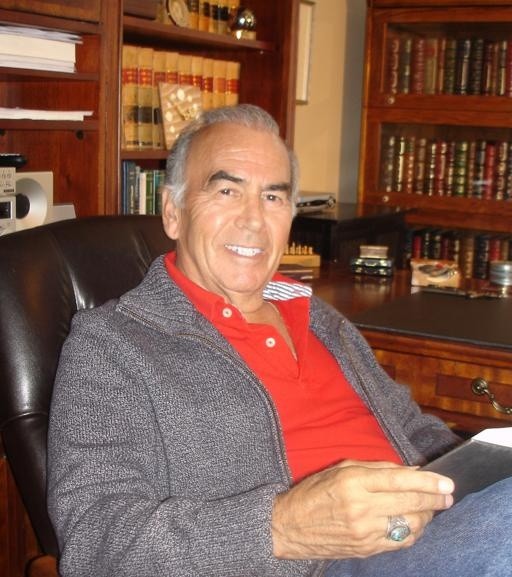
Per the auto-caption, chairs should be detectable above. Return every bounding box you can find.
[0,216,177,561]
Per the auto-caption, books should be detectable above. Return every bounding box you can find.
[382,135,512,199]
[119,45,240,149]
[401,225,512,280]
[157,0,240,38]
[415,427,512,517]
[389,35,511,98]
[120,160,166,217]
[0,34,76,63]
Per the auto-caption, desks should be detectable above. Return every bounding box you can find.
[293,202,417,281]
[300,274,512,435]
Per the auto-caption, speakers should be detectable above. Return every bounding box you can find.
[16,171,54,234]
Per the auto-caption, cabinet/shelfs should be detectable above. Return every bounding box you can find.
[2,0,112,236]
[112,2,298,234]
[361,2,512,292]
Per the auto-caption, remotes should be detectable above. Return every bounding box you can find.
[0,153,27,166]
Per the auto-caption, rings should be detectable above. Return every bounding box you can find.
[386,515,410,542]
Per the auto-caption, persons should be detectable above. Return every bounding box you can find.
[45,104,512,577]
